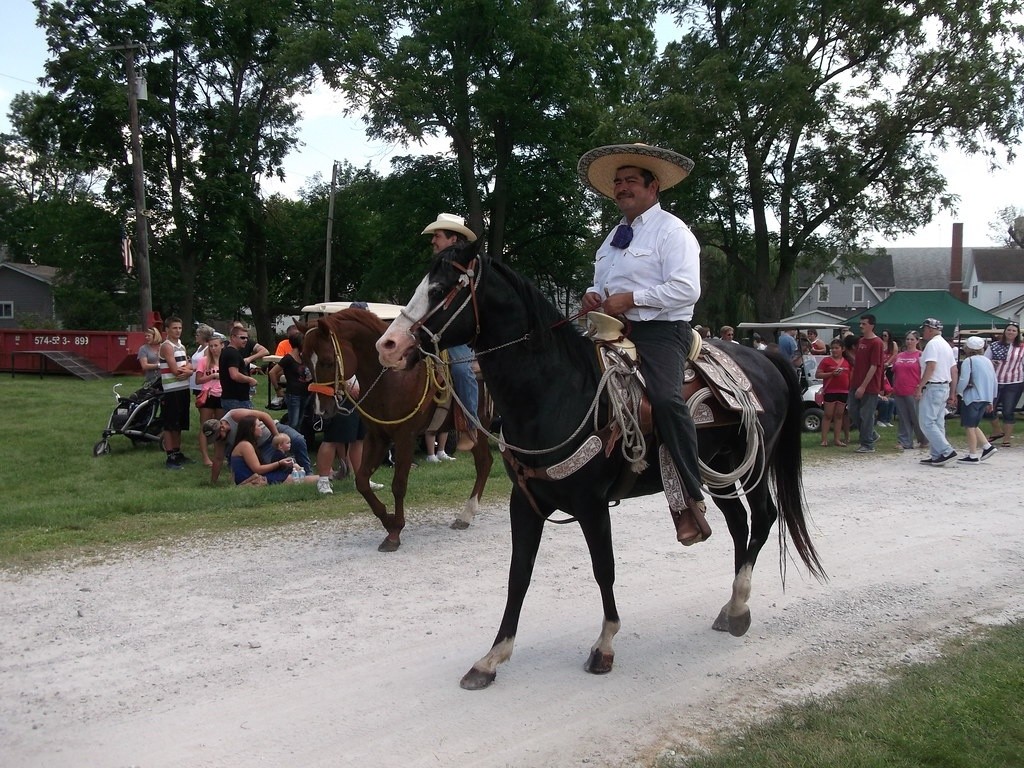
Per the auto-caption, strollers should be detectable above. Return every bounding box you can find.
[93,374,166,456]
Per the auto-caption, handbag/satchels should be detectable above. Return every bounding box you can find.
[815,387,825,407]
[238,473,267,488]
[195,387,211,408]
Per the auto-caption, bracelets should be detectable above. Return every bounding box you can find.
[157,362,161,368]
[277,460,281,468]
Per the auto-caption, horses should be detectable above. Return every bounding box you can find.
[373,234,832,692]
[291,305,497,556]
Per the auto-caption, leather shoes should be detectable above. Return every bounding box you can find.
[677,503,706,542]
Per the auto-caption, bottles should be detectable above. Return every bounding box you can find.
[299,467,306,483]
[291,467,300,486]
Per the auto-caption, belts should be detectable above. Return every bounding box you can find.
[927,381,949,384]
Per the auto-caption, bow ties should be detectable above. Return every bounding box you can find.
[609,224,633,249]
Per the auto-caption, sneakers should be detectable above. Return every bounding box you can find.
[931,449,959,466]
[354,478,385,492]
[980,445,998,461]
[317,477,334,494]
[920,458,931,465]
[957,455,979,465]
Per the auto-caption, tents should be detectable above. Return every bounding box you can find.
[834,290,1020,340]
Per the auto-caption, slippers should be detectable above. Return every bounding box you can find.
[1002,442,1011,447]
[987,432,1005,442]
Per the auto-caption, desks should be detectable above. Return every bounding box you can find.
[263,355,284,410]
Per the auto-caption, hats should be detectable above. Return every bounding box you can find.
[919,317,944,331]
[577,142,696,200]
[966,336,985,351]
[421,213,477,242]
[202,419,221,444]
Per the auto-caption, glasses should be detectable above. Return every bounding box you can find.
[235,336,248,339]
[727,334,734,336]
[808,332,813,335]
[706,336,713,339]
[882,335,886,337]
[1008,321,1019,326]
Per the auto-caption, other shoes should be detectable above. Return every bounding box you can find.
[176,452,197,464]
[853,444,875,452]
[877,420,887,427]
[820,440,828,447]
[333,457,348,481]
[166,454,183,470]
[920,442,929,447]
[885,422,894,427]
[896,443,914,449]
[425,454,442,463]
[834,441,846,446]
[437,452,456,461]
[856,430,880,444]
[456,428,478,451]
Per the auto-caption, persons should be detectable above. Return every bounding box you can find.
[694,313,1024,466]
[575,142,707,541]
[424,430,457,463]
[420,212,480,453]
[137,301,386,496]
